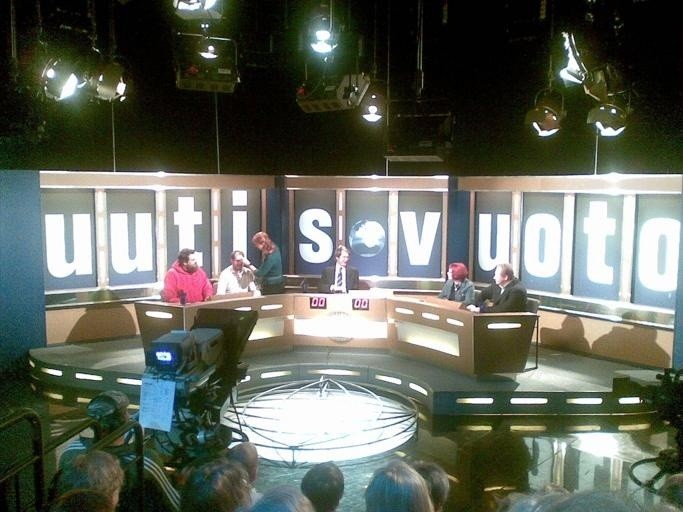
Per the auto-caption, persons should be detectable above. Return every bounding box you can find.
[213,251,256,296]
[47,390,180,511]
[317,245,359,293]
[436,263,474,305]
[180,442,682,510]
[466,263,529,313]
[159,247,212,304]
[238,232,285,295]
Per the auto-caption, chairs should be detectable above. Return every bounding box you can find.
[524,298,542,370]
[212,282,219,295]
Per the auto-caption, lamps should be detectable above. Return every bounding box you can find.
[558,18,629,94]
[363,95,384,121]
[172,1,225,26]
[305,2,343,57]
[527,87,567,137]
[298,71,372,112]
[173,32,240,92]
[586,99,635,138]
[87,41,129,100]
[29,37,79,102]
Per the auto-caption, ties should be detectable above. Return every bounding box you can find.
[337,268,343,293]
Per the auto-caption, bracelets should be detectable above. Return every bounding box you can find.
[246,263,251,268]
